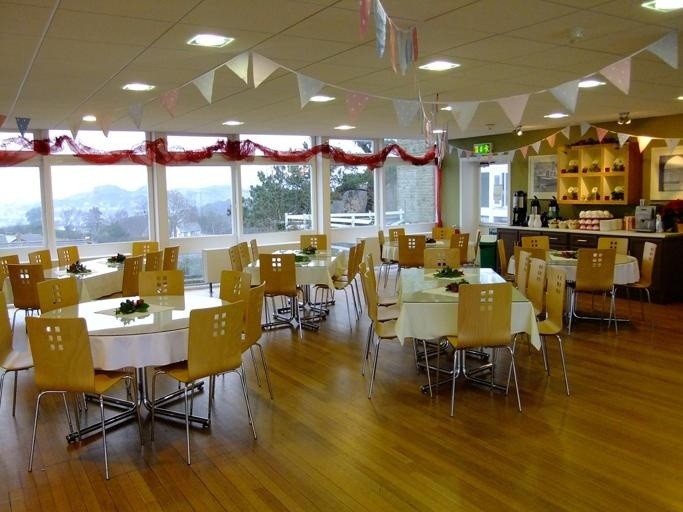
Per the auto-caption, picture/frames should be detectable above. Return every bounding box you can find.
[650,146,683,201]
[526,155,557,199]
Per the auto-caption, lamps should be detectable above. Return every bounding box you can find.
[618,112,631,124]
[512,125,523,136]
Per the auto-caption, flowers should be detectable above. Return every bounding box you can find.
[292,246,318,262]
[65,260,92,275]
[110,255,125,265]
[568,158,625,200]
[434,262,463,276]
[445,279,469,291]
[427,236,437,244]
[115,298,148,313]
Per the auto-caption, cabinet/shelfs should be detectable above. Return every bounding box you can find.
[629,237,682,305]
[557,141,642,205]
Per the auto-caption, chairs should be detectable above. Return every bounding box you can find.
[434,281,523,416]
[565,248,615,336]
[93,246,181,300]
[577,237,626,311]
[205,282,271,420]
[28,250,51,269]
[37,277,135,418]
[132,242,158,254]
[507,250,574,399]
[378,230,549,294]
[134,266,187,388]
[23,315,144,478]
[360,252,435,401]
[1,294,85,431]
[231,238,289,328]
[1,256,41,317]
[7,264,45,336]
[151,298,261,465]
[56,245,80,267]
[602,241,658,330]
[311,241,368,333]
[193,270,249,426]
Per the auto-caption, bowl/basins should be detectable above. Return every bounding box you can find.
[558,223,577,229]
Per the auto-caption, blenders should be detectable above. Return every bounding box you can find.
[511,190,560,226]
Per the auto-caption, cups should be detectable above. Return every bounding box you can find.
[579,210,612,230]
